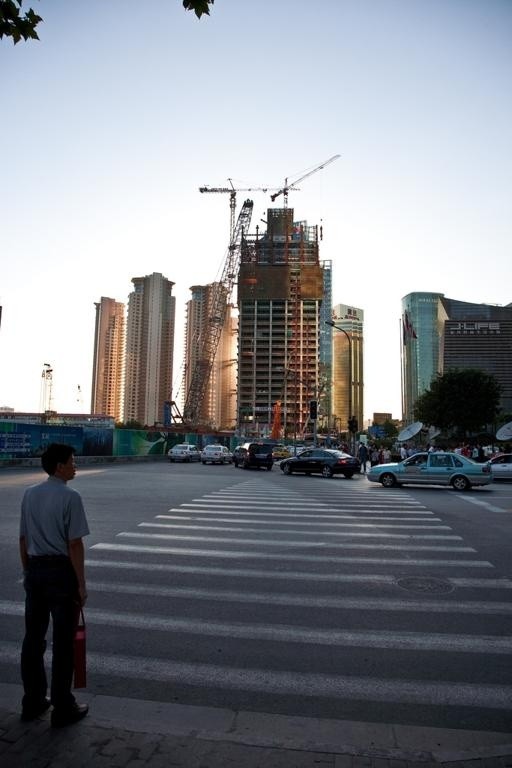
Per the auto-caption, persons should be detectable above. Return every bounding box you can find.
[328,440,488,473]
[19,444,91,728]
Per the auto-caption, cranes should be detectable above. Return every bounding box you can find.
[163,154,341,433]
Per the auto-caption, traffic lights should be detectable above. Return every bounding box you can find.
[310,402,317,419]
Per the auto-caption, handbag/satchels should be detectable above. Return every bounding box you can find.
[74,607,87,688]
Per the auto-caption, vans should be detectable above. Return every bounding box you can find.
[235,442,272,470]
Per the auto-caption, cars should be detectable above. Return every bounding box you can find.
[280,448,361,478]
[272,447,293,461]
[367,447,512,491]
[167,442,239,464]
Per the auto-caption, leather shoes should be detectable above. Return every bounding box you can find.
[50,702,89,728]
[20,697,53,722]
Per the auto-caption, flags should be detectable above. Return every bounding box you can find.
[402,311,417,346]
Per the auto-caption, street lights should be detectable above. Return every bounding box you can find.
[325,322,354,442]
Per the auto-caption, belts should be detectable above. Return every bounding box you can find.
[26,555,68,564]
